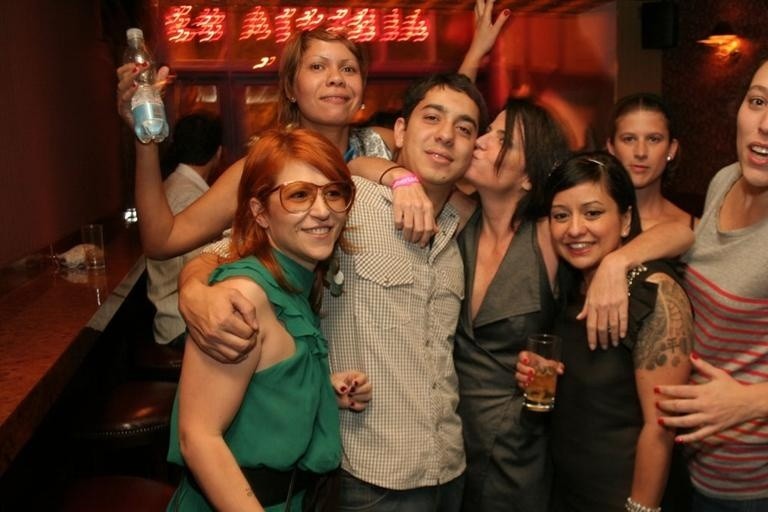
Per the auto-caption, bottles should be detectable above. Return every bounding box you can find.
[125,27,171,146]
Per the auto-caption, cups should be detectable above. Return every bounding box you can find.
[78,225,107,270]
[523,334,566,410]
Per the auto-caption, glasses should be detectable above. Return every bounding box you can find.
[259,180,355,214]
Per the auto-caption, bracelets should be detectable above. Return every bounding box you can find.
[373,165,409,178]
[387,176,425,185]
[622,496,664,512]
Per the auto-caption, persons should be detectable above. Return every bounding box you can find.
[161,142,371,511]
[148,109,237,369]
[176,69,489,512]
[652,45,768,511]
[345,100,696,510]
[113,1,512,261]
[512,155,699,511]
[601,87,703,241]
